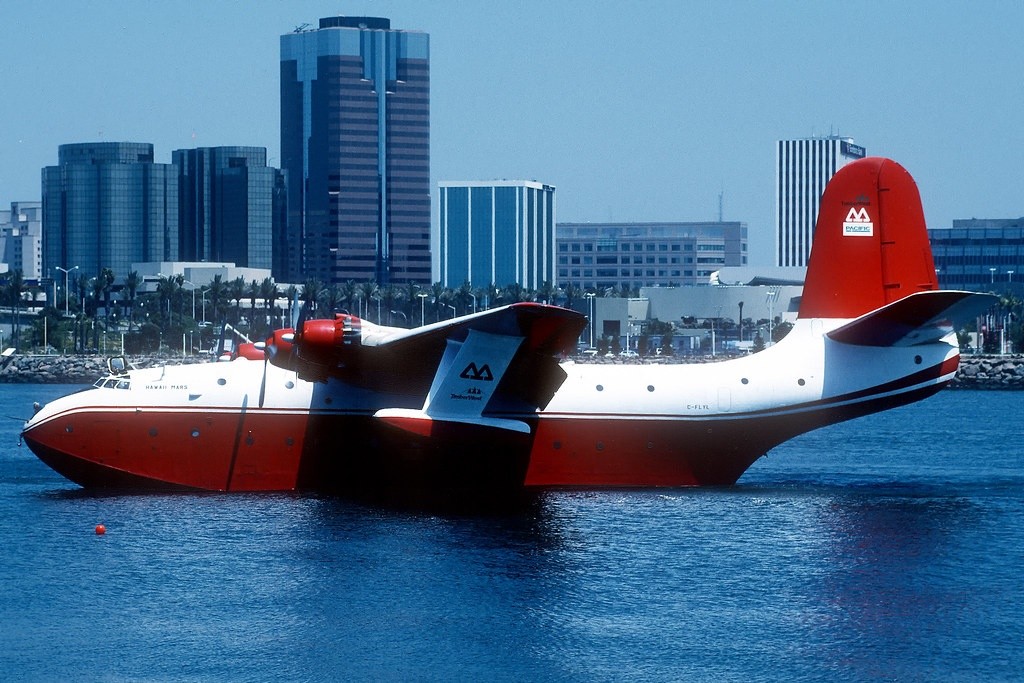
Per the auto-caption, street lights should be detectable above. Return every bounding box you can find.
[52,263,1016,360]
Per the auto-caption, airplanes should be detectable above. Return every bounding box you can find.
[18,155,978,500]
